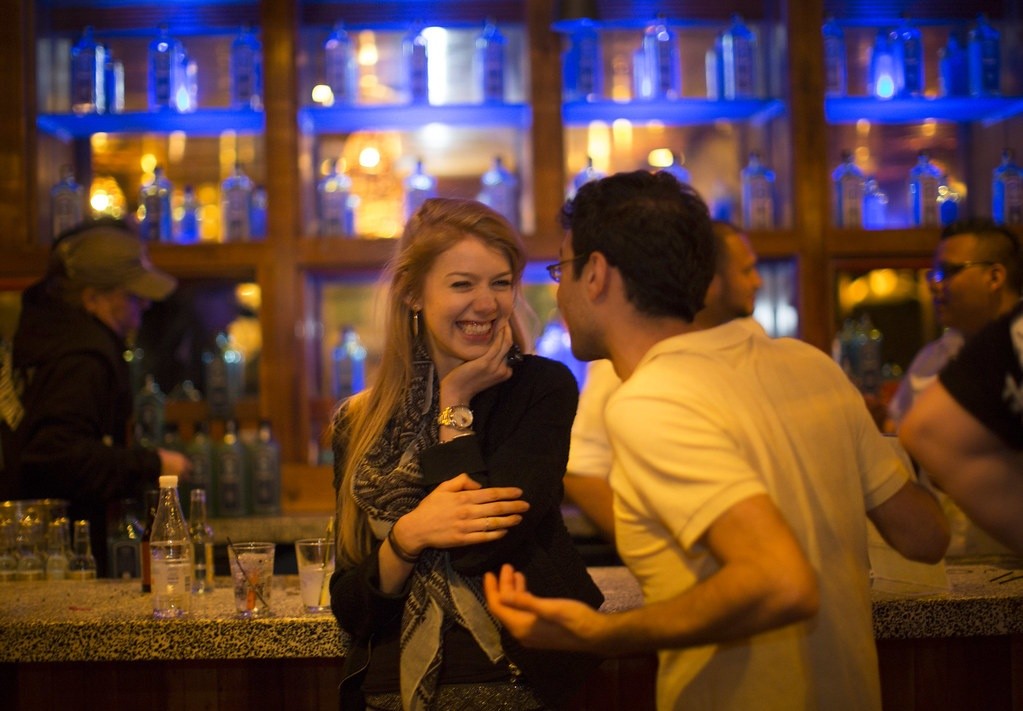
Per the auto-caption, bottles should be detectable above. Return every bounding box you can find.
[107,475,214,621]
[328,326,368,400]
[136,331,281,518]
[47,150,781,245]
[70,14,786,115]
[0,508,97,582]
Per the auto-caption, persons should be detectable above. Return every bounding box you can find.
[564,220,762,545]
[329,196,605,711]
[483,169,953,711]
[884,216,1023,555]
[0,219,195,577]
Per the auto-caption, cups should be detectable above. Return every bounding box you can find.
[226,542,276,619]
[295,537,336,615]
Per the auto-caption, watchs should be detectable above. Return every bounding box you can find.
[436,403,474,429]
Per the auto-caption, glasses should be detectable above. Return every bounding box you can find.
[927,258,993,282]
[547,252,586,284]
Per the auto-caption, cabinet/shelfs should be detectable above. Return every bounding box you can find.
[0,1,1023,510]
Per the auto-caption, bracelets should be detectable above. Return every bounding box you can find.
[387,520,420,562]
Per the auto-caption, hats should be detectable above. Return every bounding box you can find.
[47,227,176,299]
